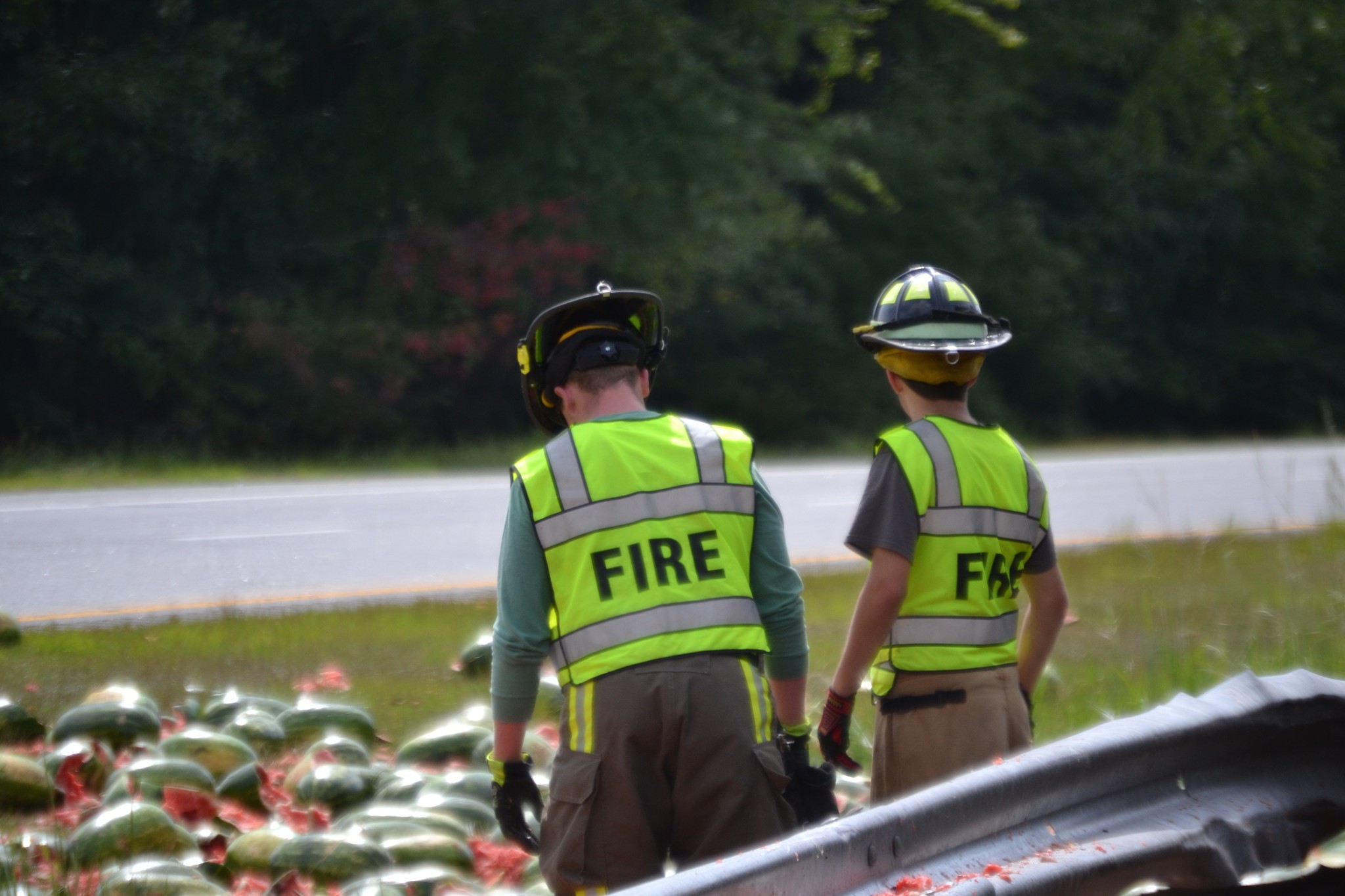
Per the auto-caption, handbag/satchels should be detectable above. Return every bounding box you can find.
[777,733,840,828]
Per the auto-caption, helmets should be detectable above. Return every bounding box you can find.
[853,265,1012,364]
[517,281,669,436]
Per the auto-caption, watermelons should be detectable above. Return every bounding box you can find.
[0,630,849,896]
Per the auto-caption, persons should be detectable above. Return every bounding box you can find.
[486,289,812,895]
[816,266,1070,805]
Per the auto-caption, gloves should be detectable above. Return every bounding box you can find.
[817,687,864,778]
[1019,685,1035,728]
[486,750,545,856]
[775,718,811,767]
